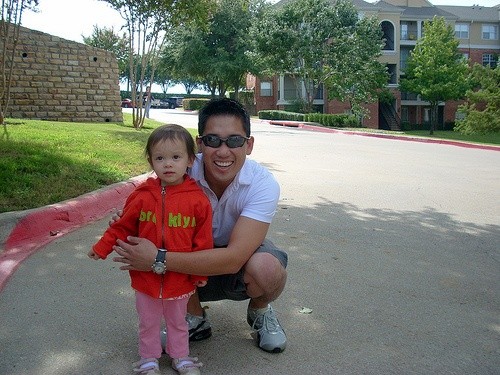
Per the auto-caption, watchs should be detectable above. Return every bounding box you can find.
[151,249,167,274]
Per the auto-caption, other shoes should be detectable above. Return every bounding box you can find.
[133,358,162,375]
[172,357,203,375]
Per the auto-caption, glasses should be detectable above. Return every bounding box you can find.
[198,134,248,149]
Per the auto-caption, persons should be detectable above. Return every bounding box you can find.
[87,124,214,375]
[109,98,288,354]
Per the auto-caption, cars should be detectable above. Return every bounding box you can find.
[122,95,184,109]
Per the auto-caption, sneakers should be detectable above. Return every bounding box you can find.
[247,303,287,353]
[161,305,211,354]
